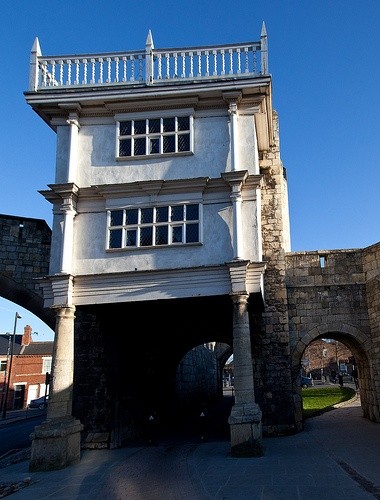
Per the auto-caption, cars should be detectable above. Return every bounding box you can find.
[29,395,50,411]
[300,375,314,389]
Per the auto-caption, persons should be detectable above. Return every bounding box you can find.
[351,366,359,390]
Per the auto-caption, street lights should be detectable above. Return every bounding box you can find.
[2,312,24,419]
